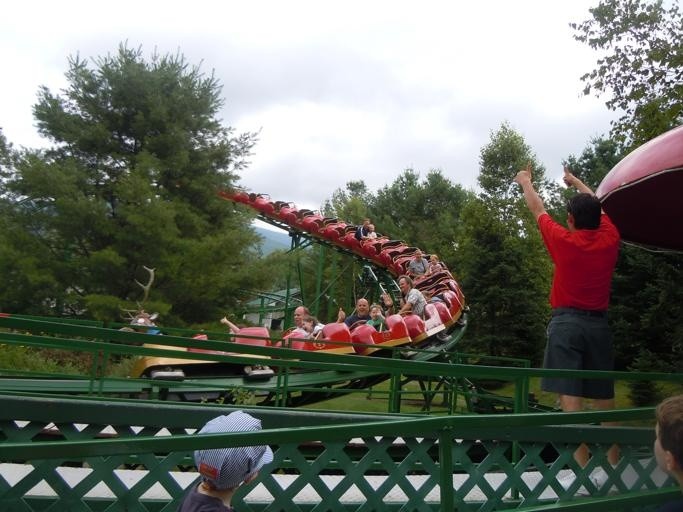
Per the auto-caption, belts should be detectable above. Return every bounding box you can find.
[552,307,604,317]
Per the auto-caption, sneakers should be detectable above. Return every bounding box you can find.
[555,465,617,495]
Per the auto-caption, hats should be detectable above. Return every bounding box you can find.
[194,410,274,490]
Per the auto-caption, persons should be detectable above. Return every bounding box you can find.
[177,411,274,510]
[654,397,679,489]
[221,219,440,338]
[515,162,619,496]
